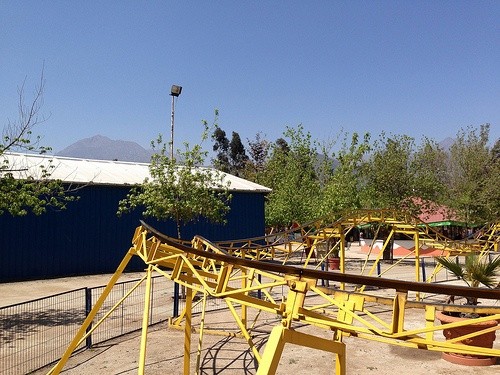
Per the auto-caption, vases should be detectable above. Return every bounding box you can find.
[328,247,340,270]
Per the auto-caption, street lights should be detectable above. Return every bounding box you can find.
[168,82,186,272]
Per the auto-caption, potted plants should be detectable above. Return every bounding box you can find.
[430,253,499,367]
